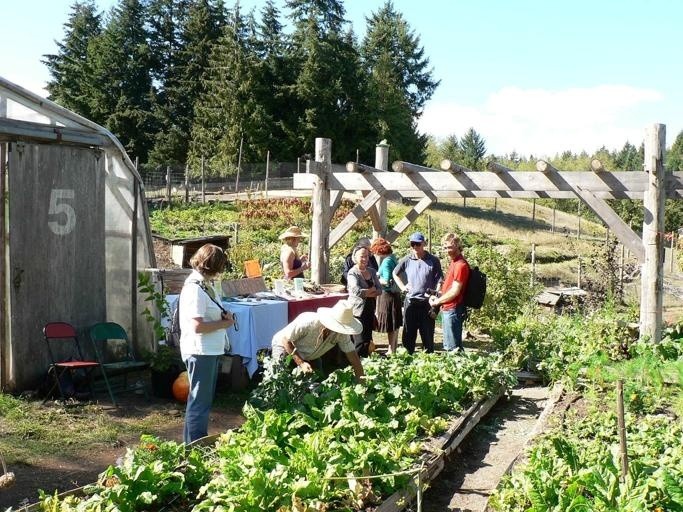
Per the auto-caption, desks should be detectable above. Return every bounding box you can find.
[221,289,349,379]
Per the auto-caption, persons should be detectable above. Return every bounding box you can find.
[278,226,309,280]
[391,233,443,356]
[370,237,403,354]
[345,246,382,359]
[270,298,365,381]
[427,233,470,350]
[340,236,380,286]
[177,243,236,447]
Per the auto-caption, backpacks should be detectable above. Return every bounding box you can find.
[463,259,486,309]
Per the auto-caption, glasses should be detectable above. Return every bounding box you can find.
[411,242,421,247]
[233,313,239,331]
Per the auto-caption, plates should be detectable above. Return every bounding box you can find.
[301,287,324,294]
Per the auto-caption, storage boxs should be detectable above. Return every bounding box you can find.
[216,353,243,393]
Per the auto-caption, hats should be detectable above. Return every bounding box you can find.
[279,226,305,239]
[317,300,363,335]
[410,232,424,242]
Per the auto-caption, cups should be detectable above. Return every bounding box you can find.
[293,277,304,301]
[274,280,284,296]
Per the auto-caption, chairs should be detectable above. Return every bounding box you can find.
[42,322,100,413]
[88,322,152,407]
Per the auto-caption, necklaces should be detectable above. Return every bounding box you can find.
[359,268,367,273]
